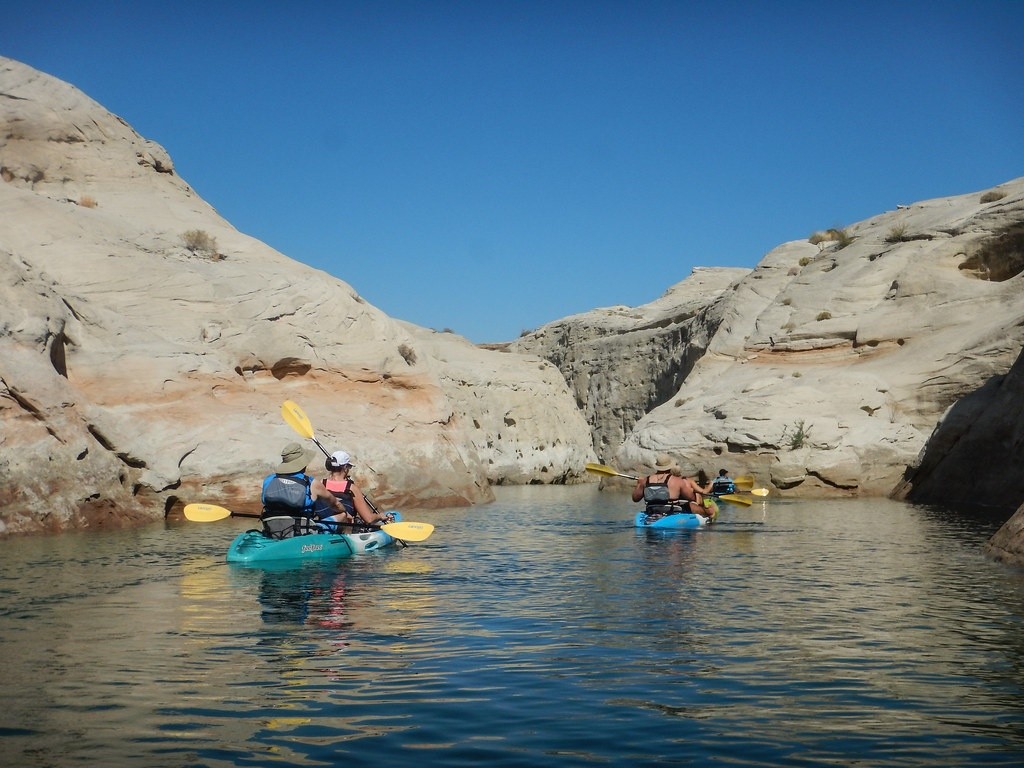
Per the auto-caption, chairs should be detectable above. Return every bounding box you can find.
[261,516,319,538]
[647,505,682,514]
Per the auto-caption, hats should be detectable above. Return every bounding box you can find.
[718,469,728,476]
[330,450,355,467]
[275,443,316,474]
[653,453,676,471]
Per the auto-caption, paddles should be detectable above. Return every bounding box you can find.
[281,399,408,547]
[585,462,752,509]
[696,474,754,489]
[737,488,769,497]
[182,502,435,542]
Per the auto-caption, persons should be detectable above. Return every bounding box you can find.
[710,469,741,496]
[261,442,354,535]
[632,454,718,519]
[321,451,389,533]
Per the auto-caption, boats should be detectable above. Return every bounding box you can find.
[226,511,401,569]
[634,510,715,531]
